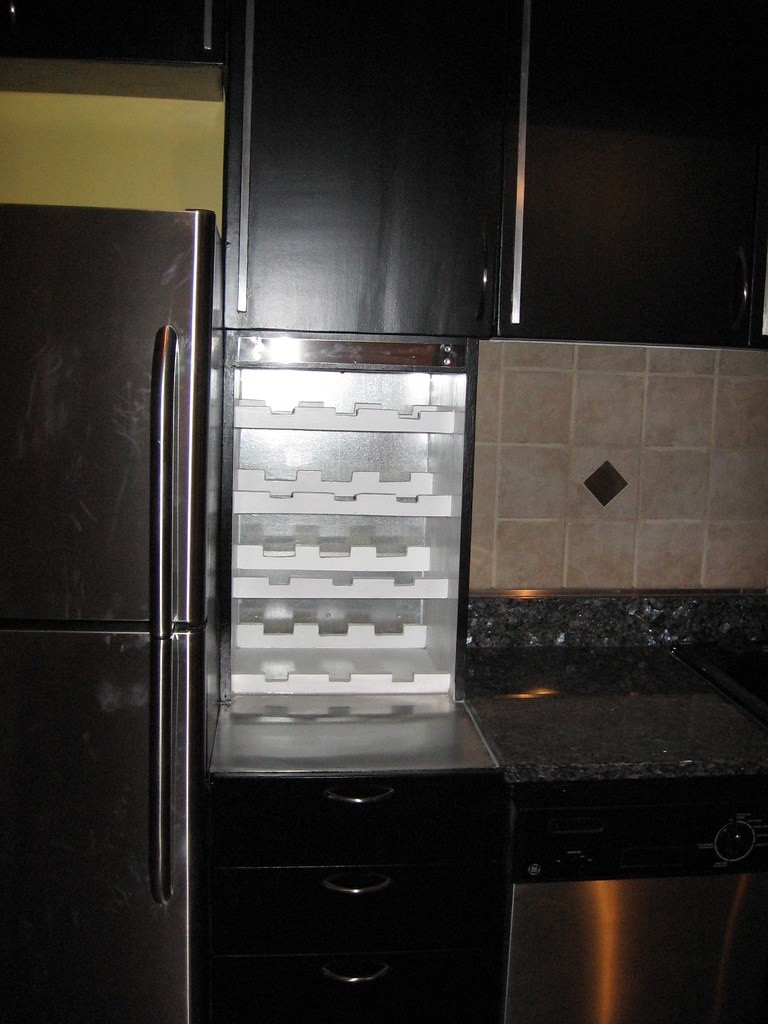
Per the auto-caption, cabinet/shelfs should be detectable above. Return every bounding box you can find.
[199,777,514,1023]
[0,0,768,346]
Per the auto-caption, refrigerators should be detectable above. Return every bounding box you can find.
[0,203,219,1024]
